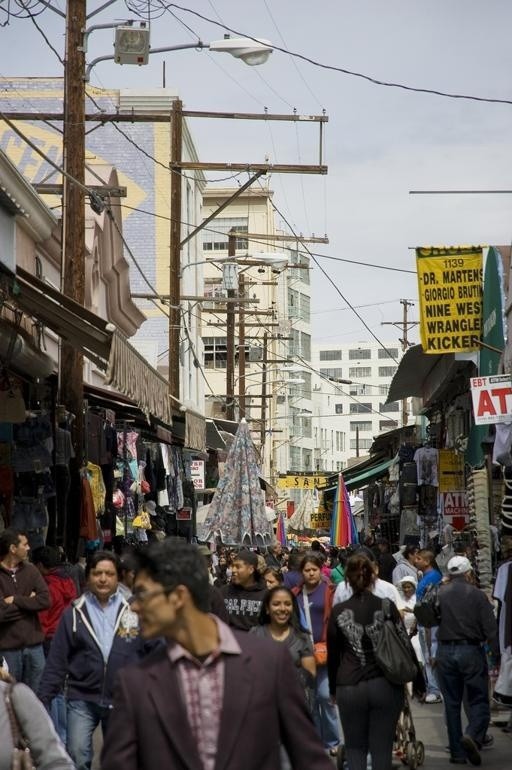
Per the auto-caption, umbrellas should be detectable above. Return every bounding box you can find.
[327,470,364,550]
[204,416,284,556]
[288,486,319,534]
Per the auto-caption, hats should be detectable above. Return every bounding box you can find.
[448,556,473,575]
[399,576,416,588]
[145,500,156,516]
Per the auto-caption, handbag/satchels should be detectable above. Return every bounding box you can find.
[133,512,152,529]
[130,471,150,496]
[313,641,326,664]
[12,747,36,770]
[364,599,417,686]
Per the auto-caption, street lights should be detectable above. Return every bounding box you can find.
[225,365,307,424]
[61,37,276,559]
[167,253,290,462]
[238,377,306,424]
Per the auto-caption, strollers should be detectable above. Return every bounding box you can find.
[335,684,424,770]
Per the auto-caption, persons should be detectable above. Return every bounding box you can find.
[1,529,442,770]
[435,555,501,768]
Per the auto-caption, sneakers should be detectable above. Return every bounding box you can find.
[445,734,493,765]
[425,693,442,703]
